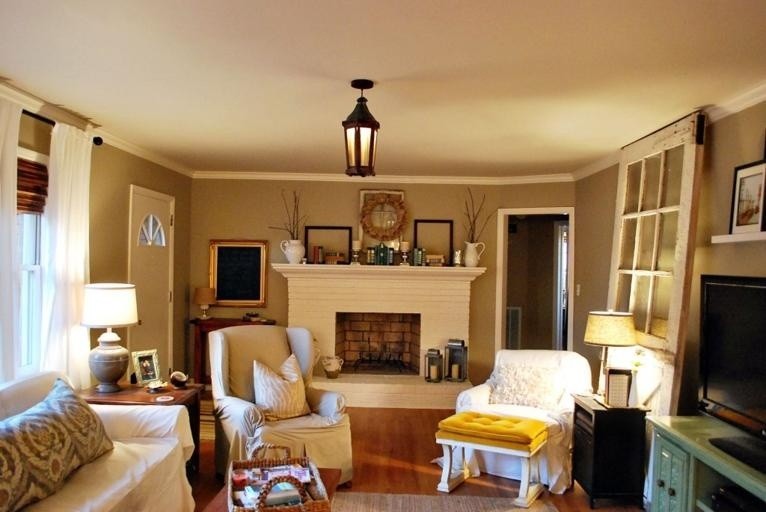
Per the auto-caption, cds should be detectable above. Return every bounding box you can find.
[156,395,174,402]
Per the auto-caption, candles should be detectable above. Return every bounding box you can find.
[429,363,439,381]
[351,240,361,251]
[449,362,459,380]
[400,241,410,252]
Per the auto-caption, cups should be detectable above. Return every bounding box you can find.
[313,246,324,263]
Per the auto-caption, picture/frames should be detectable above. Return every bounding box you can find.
[130,347,163,386]
[206,236,271,309]
[412,217,454,267]
[303,223,354,265]
[726,158,766,235]
[357,188,406,255]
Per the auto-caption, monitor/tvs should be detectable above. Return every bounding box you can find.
[697,274,766,475]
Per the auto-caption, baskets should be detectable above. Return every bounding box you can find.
[226,443,330,512]
[320,357,345,379]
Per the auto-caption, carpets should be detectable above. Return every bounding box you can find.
[325,487,562,511]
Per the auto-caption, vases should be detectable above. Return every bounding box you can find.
[463,240,487,267]
[278,239,307,264]
[321,355,346,381]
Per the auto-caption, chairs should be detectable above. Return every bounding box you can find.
[204,320,355,494]
[453,346,598,497]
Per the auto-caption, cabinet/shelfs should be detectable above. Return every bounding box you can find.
[566,388,652,508]
[639,408,766,511]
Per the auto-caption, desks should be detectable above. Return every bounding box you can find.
[185,314,277,400]
[74,380,206,480]
[202,460,344,510]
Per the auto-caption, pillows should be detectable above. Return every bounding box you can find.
[251,355,314,421]
[0,375,112,510]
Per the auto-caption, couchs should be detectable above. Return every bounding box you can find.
[0,368,201,510]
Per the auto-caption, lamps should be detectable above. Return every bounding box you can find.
[192,286,217,321]
[339,77,381,179]
[580,307,640,409]
[81,280,141,396]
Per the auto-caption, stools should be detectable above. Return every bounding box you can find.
[432,409,554,508]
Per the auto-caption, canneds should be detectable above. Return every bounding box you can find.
[366,247,375,265]
[313,245,323,264]
[389,248,393,264]
[413,248,426,266]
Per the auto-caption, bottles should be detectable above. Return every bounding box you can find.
[322,353,343,378]
[453,249,464,265]
